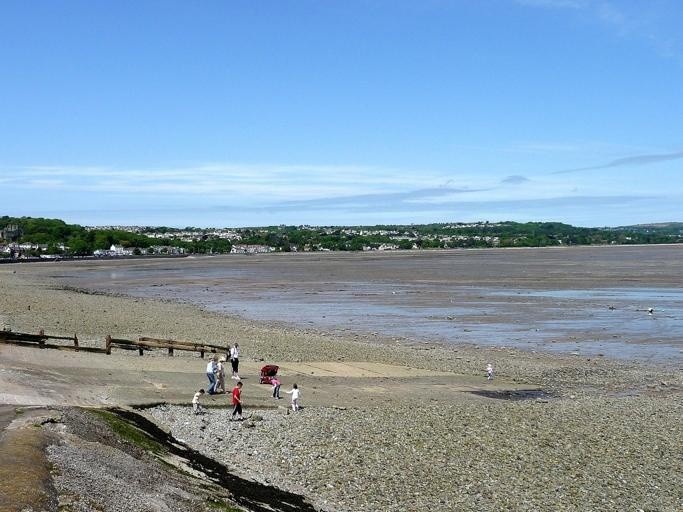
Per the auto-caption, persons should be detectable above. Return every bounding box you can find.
[228,381,245,421]
[268,378,280,400]
[205,355,218,395]
[229,342,241,380]
[191,389,205,412]
[284,382,301,411]
[485,363,493,380]
[213,359,229,394]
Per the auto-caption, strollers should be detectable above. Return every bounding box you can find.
[260,364,279,384]
